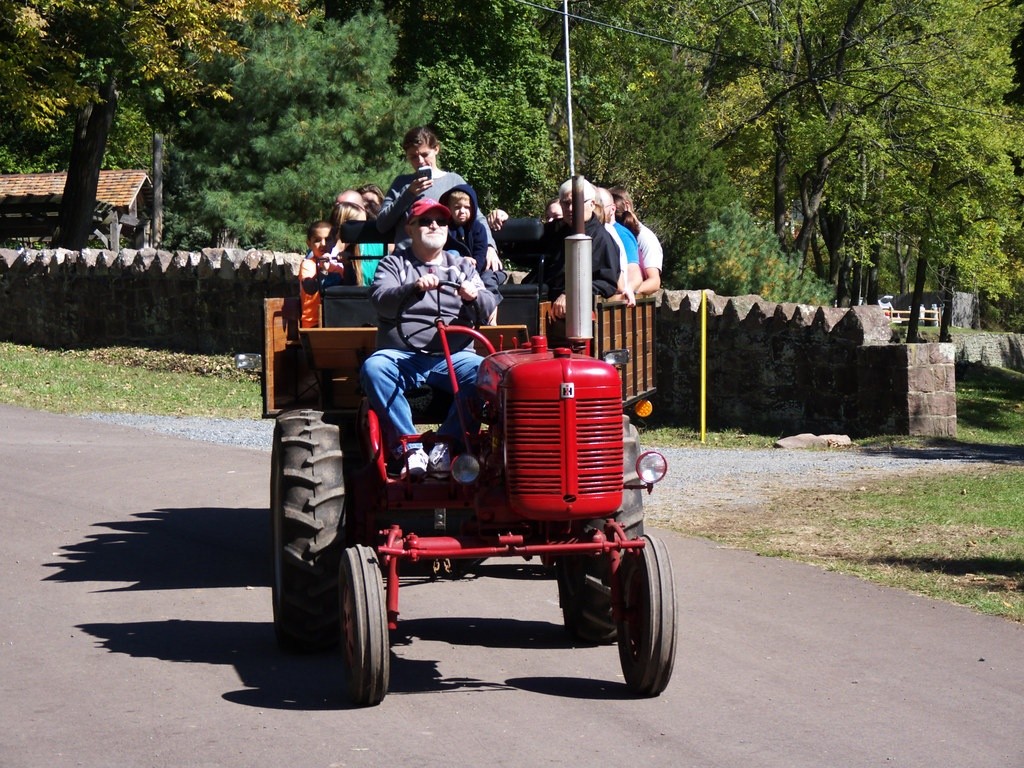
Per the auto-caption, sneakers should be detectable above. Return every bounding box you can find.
[428,442,452,479]
[400,447,430,481]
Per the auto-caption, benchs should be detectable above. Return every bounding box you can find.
[314,217,544,338]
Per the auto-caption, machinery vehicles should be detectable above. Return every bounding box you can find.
[256,172,683,707]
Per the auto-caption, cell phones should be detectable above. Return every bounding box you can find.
[416,166,432,181]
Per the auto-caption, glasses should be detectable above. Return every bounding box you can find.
[410,217,449,226]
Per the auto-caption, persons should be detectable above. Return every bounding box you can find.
[434,184,507,327]
[361,198,497,484]
[378,126,505,274]
[486,175,622,348]
[307,177,665,308]
[299,221,348,328]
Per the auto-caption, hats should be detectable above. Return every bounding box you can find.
[407,197,452,224]
